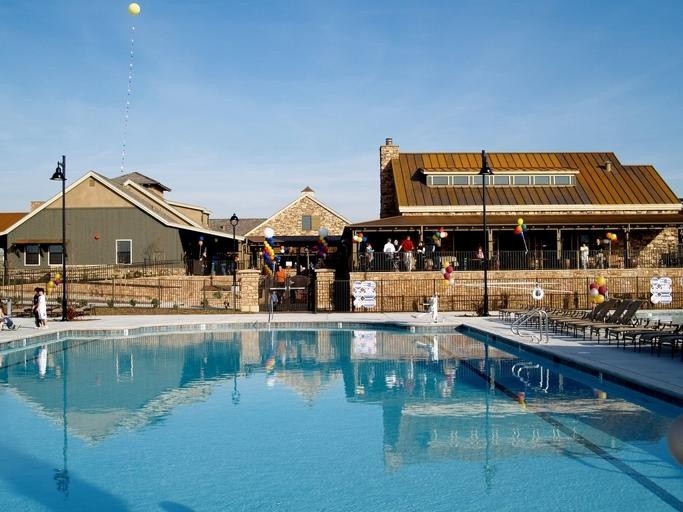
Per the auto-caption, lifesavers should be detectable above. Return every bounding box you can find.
[532,287,544,300]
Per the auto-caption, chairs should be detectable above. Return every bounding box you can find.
[550,299,645,339]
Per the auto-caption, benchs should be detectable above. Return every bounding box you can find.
[496,302,588,332]
[589,316,683,361]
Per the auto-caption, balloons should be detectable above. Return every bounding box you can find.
[589,276,607,303]
[603,233,619,244]
[263,228,330,280]
[128,3,140,16]
[353,233,367,242]
[231,254,239,262]
[433,227,448,241]
[46,272,63,295]
[215,238,218,242]
[514,218,527,234]
[199,236,204,246]
[441,261,454,286]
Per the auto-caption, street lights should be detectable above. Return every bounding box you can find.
[478,149,494,317]
[230,213,239,309]
[49,155,68,322]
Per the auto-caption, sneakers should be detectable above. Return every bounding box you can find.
[10,324,19,330]
[33,325,47,330]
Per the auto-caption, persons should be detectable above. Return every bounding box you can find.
[580,238,604,269]
[32,288,47,329]
[474,246,498,269]
[605,287,608,300]
[0,305,21,331]
[365,237,441,272]
[270,266,285,311]
[429,292,438,322]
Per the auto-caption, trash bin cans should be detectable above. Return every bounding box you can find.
[424,258,433,271]
[617,257,625,268]
[563,259,571,269]
[361,257,369,271]
[564,292,573,310]
